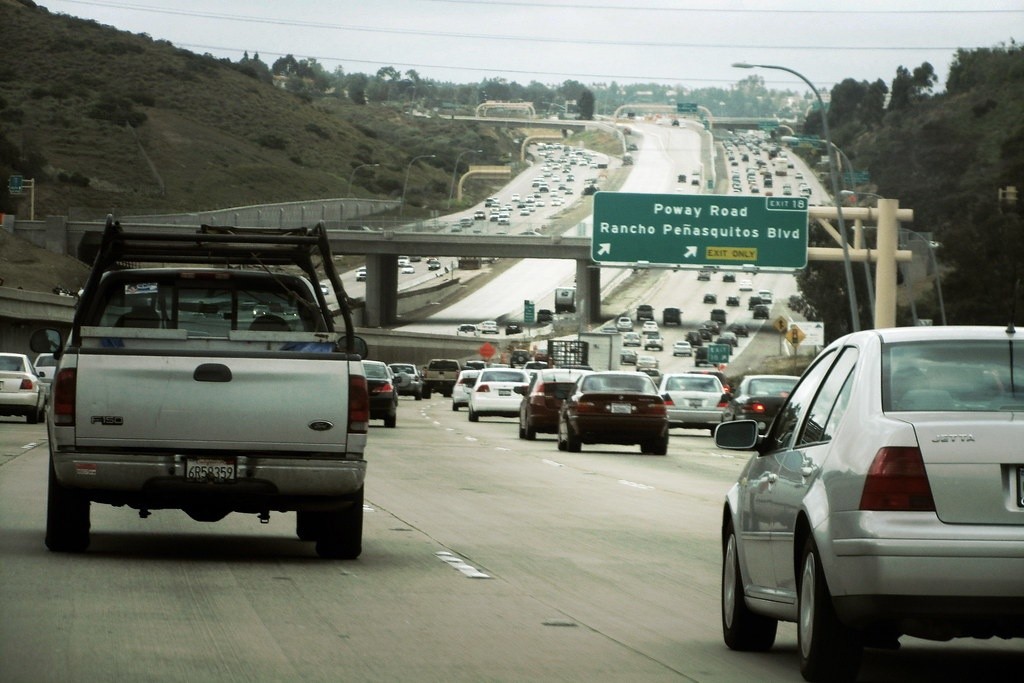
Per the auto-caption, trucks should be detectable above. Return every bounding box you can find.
[579,331,622,372]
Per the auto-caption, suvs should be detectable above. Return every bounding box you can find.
[387,363,423,400]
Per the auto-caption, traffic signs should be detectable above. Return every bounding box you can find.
[591,190,808,270]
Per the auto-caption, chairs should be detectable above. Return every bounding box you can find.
[113,311,159,329]
[247,315,292,332]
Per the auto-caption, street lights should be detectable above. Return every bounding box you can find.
[781,136,876,319]
[729,62,862,331]
[399,154,436,215]
[347,163,380,198]
[839,190,950,325]
[448,150,483,210]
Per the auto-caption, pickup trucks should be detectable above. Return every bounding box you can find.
[44,213,369,559]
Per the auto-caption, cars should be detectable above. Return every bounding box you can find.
[0,352,50,424]
[537,309,554,322]
[32,352,58,390]
[505,322,523,335]
[677,133,815,200]
[602,264,778,396]
[519,368,595,440]
[457,320,500,336]
[359,359,402,428]
[558,371,670,456]
[422,340,592,422]
[319,139,609,305]
[713,325,1023,683]
[720,373,802,434]
[656,373,736,440]
